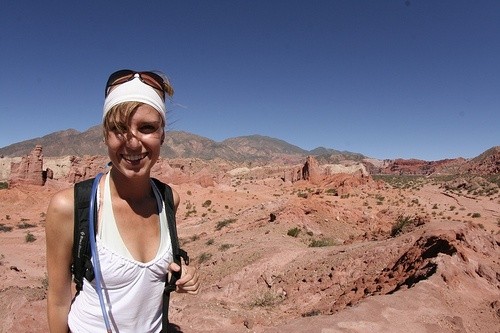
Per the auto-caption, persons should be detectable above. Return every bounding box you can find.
[44,69,201,333]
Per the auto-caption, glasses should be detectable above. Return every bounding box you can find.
[105,69,166,104]
